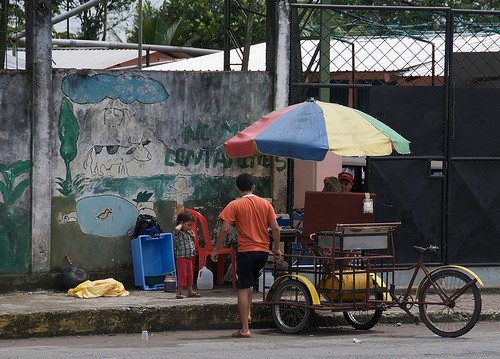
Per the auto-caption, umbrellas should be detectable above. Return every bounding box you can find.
[223,95,414,192]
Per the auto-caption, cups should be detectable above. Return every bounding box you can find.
[142,331,148,340]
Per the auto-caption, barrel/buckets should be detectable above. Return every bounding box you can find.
[164,272,176,293]
[258,270,274,293]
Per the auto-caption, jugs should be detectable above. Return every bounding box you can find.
[196,266,213,289]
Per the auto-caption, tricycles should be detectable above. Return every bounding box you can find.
[254,221,482,337]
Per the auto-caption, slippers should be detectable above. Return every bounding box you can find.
[231,328,252,338]
[176,292,183,299]
[235,314,251,324]
[186,292,201,298]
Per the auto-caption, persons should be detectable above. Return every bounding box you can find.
[210,172,281,339]
[321,171,354,192]
[174,210,202,299]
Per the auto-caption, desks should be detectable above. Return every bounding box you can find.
[263,229,298,302]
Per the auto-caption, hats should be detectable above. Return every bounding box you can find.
[338,172,353,183]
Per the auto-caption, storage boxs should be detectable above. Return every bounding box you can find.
[276,213,291,229]
[130,233,178,290]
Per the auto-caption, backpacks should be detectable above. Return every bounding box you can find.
[131,214,163,240]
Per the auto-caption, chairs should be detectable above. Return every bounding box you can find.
[184,208,236,289]
